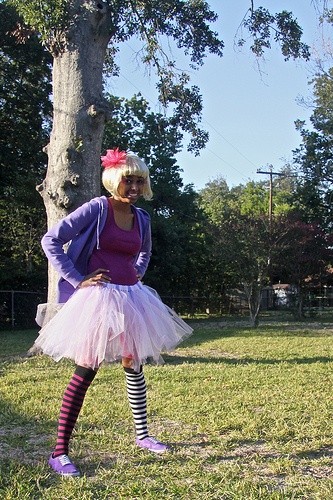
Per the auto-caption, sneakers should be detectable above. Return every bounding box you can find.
[49,452,80,477]
[137,437,170,453]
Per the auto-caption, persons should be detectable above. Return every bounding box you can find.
[28,148,195,477]
